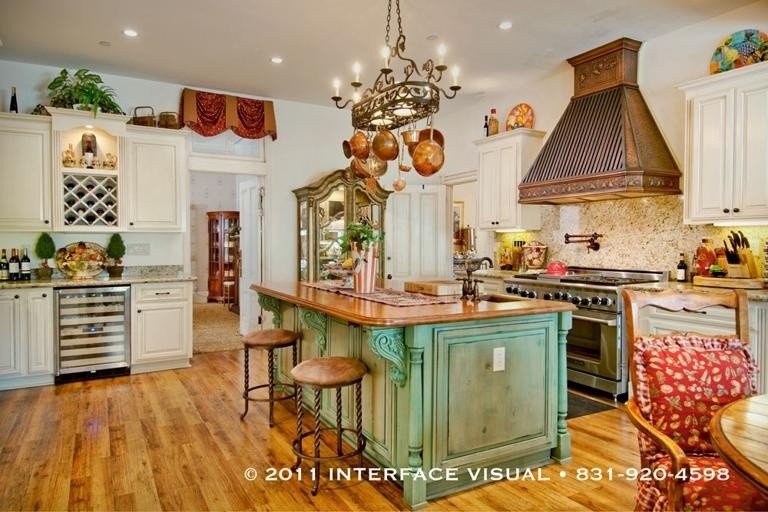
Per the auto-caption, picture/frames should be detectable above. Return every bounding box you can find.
[452,201,465,245]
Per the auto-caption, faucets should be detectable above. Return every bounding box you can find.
[456,257,494,301]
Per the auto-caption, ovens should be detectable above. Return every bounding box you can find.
[567,309,622,398]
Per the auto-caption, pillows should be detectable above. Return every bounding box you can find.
[628,331,761,463]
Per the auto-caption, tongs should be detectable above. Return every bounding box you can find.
[519,85,683,206]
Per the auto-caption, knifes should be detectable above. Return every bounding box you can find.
[721,229,750,264]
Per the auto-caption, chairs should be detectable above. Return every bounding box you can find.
[621,284,760,511]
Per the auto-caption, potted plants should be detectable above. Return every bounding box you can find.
[105,232,127,277]
[35,233,56,280]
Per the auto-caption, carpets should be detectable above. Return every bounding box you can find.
[565,387,617,419]
[191,301,247,356]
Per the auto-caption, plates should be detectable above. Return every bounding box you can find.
[506,103,535,130]
[57,242,108,278]
[710,28,768,75]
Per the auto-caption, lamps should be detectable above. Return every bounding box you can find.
[330,0,461,132]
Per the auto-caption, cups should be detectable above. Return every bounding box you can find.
[495,242,512,270]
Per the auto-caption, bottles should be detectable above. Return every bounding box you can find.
[9,86,18,114]
[677,252,687,281]
[689,252,702,282]
[488,109,499,136]
[65,182,116,225]
[511,240,525,270]
[0,247,32,281]
[481,116,488,138]
[84,140,93,168]
[699,239,716,274]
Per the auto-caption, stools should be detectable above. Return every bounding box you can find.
[237,329,302,429]
[289,355,370,495]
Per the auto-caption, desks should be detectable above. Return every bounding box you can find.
[250,282,577,511]
[710,393,768,496]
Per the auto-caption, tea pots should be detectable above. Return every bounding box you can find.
[61,142,75,167]
[102,151,116,170]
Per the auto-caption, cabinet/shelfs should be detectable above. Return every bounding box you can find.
[130,277,197,375]
[1,283,56,391]
[473,128,544,231]
[124,125,191,233]
[293,169,395,284]
[453,271,506,296]
[674,60,768,228]
[0,112,52,233]
[205,211,240,303]
[625,285,768,402]
[46,106,125,233]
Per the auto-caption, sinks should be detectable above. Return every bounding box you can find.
[478,293,531,303]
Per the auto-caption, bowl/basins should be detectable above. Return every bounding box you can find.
[523,246,547,268]
[547,262,568,272]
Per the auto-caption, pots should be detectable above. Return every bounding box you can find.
[342,110,445,179]
